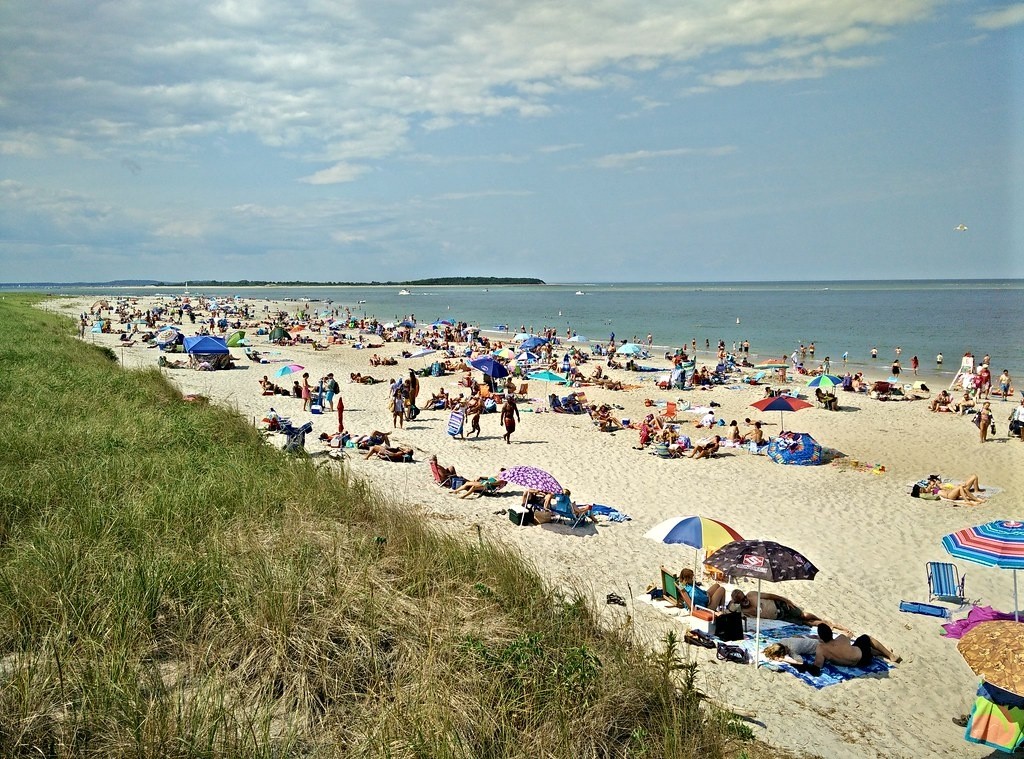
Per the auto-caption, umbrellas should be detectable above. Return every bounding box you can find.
[495,466,563,531]
[805,372,842,411]
[157,326,181,333]
[410,348,436,370]
[749,396,814,430]
[518,335,548,350]
[957,619,1024,699]
[942,519,1024,623]
[273,364,305,386]
[514,351,540,370]
[129,318,148,336]
[493,348,516,359]
[753,358,790,397]
[703,539,820,672]
[527,369,567,407]
[290,324,309,340]
[471,358,509,393]
[467,355,492,375]
[615,342,642,371]
[567,334,589,358]
[513,333,532,344]
[337,397,344,470]
[643,515,744,630]
[768,431,822,466]
[156,329,178,346]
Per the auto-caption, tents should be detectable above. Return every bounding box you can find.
[226,331,245,348]
[269,328,291,342]
[90,322,104,334]
[182,335,231,371]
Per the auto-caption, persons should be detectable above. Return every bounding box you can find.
[79,296,1024,667]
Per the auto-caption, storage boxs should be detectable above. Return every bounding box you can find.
[311,405,322,414]
[452,478,464,490]
[331,434,351,447]
[507,505,534,525]
[691,604,716,633]
[260,359,267,363]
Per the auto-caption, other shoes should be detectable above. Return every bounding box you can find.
[961,714,971,721]
[606,592,626,607]
[952,718,967,727]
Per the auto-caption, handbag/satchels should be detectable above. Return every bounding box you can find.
[1009,423,1015,431]
[709,613,744,641]
[685,629,716,649]
[716,643,748,664]
[389,400,395,412]
[534,507,552,524]
[991,423,996,435]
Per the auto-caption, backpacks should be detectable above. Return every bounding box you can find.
[329,379,339,394]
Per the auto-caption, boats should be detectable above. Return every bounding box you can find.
[398,289,411,296]
[575,290,584,296]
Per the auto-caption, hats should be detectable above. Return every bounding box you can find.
[645,581,658,594]
[795,350,799,353]
[981,364,988,369]
[328,373,333,377]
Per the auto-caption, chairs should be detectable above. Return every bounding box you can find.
[473,477,508,498]
[514,383,529,400]
[925,561,971,609]
[259,380,275,395]
[244,349,259,362]
[654,444,683,458]
[548,395,563,411]
[561,397,578,411]
[428,458,457,487]
[585,406,610,429]
[748,440,769,456]
[374,444,410,462]
[554,493,591,529]
